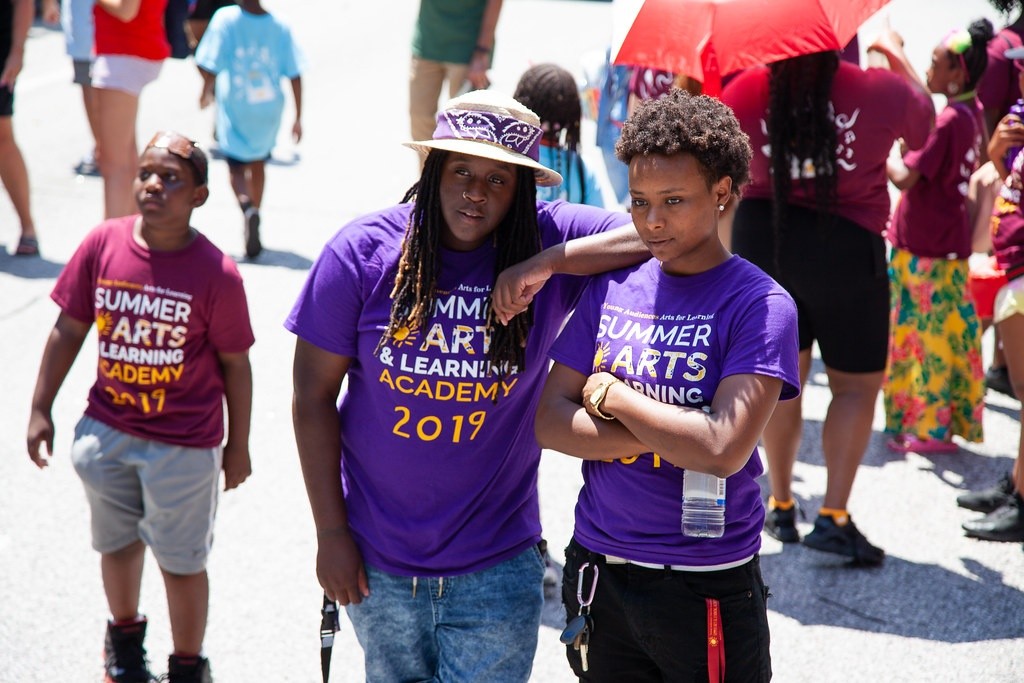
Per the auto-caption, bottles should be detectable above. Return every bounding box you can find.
[681,406,725,537]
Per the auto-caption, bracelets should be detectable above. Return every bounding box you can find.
[474,45,491,54]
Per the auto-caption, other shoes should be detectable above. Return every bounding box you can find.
[17,235,38,255]
[240,199,262,257]
[983,365,1017,398]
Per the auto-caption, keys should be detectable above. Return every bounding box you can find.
[560,615,594,672]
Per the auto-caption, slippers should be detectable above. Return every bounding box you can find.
[886,432,959,452]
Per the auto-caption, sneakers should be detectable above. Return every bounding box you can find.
[104,616,150,683]
[168,652,212,683]
[762,495,802,543]
[957,468,1024,543]
[803,513,882,567]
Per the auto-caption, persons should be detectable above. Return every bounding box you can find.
[534,88,801,683]
[183,0,302,257]
[43,0,171,220]
[0,0,38,255]
[512,48,746,208]
[719,34,937,564]
[284,90,653,683]
[27,130,256,683]
[883,19,995,452]
[409,0,503,174]
[957,0,1024,542]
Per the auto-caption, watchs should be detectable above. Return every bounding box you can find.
[589,379,619,420]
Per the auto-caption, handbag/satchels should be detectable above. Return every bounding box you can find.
[164,0,192,58]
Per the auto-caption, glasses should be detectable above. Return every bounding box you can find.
[944,25,972,83]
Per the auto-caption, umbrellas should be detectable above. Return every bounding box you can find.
[614,0,891,85]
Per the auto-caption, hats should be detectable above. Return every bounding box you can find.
[401,87,564,188]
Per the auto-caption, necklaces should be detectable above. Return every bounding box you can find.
[946,89,978,104]
